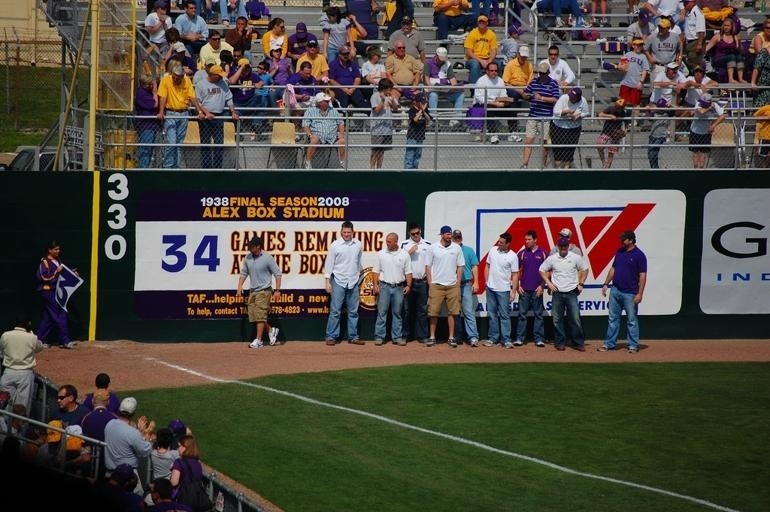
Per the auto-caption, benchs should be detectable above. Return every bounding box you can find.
[37,0,770,166]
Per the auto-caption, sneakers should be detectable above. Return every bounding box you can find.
[248,339,264,348]
[590,18,612,27]
[488,135,500,144]
[375,338,586,352]
[326,340,337,345]
[508,136,522,143]
[628,349,639,353]
[268,327,279,346]
[597,345,609,352]
[448,120,461,129]
[349,340,365,345]
[59,341,78,348]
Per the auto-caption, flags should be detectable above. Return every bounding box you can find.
[54,263,84,312]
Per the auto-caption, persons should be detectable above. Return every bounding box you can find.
[0,316,203,512]
[134,1,770,170]
[597,231,647,354]
[37,239,79,349]
[373,222,588,351]
[236,236,282,348]
[324,221,365,346]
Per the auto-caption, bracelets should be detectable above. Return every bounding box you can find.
[604,284,608,285]
[275,289,280,292]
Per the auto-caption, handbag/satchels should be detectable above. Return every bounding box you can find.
[581,30,599,40]
[176,479,213,512]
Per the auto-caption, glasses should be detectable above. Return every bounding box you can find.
[55,396,66,400]
[411,231,420,236]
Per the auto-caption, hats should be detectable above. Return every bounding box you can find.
[439,226,452,235]
[46,420,63,444]
[119,397,137,416]
[558,228,572,237]
[154,1,449,104]
[615,11,712,112]
[620,231,635,240]
[169,419,186,435]
[65,425,85,453]
[559,238,568,247]
[94,388,111,401]
[245,237,263,247]
[453,230,461,238]
[477,15,582,100]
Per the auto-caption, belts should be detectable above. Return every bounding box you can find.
[166,108,188,112]
[381,281,402,288]
